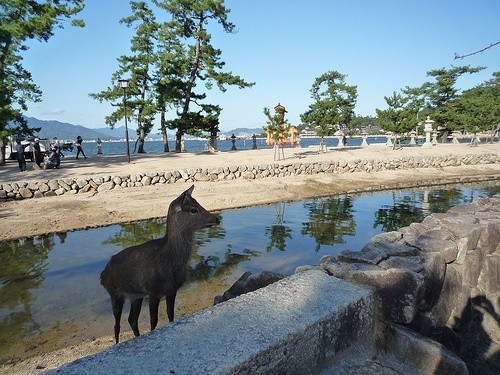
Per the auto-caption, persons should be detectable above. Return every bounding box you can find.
[15,136,65,172]
[75,136,88,160]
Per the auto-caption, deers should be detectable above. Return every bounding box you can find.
[100,185,220,342]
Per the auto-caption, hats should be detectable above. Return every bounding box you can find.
[34,138,39,142]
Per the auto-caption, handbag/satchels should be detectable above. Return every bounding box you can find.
[76,143,80,146]
